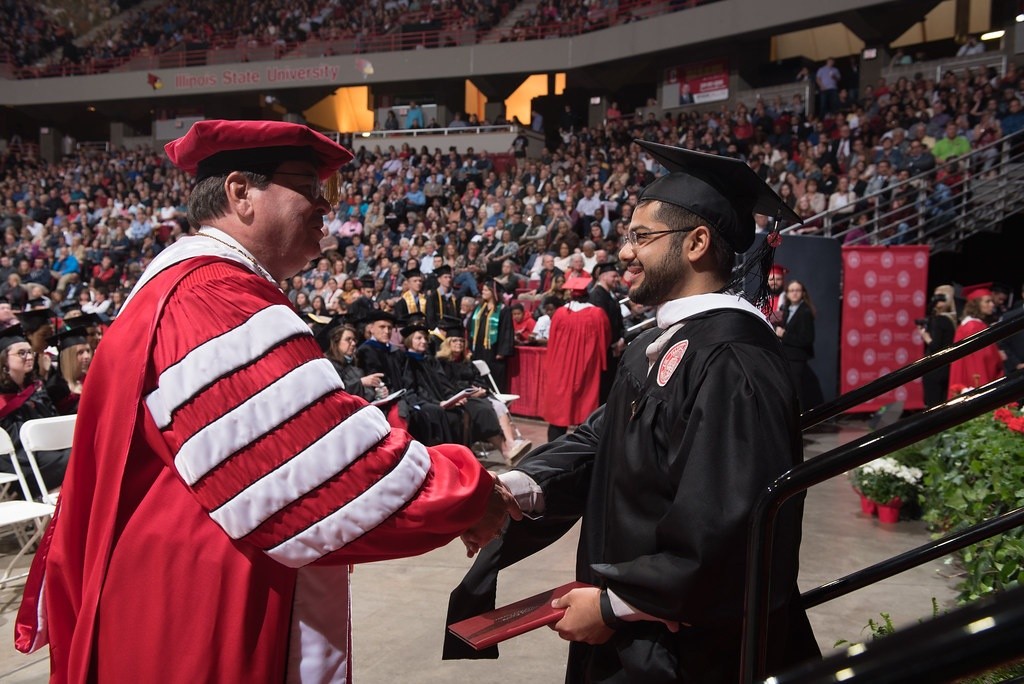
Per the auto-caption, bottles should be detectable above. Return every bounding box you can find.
[376,382,385,401]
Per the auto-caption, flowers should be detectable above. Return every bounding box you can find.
[849,454,922,506]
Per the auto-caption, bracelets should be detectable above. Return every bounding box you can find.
[600,586,647,631]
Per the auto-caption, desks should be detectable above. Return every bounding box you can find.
[501,345,550,422]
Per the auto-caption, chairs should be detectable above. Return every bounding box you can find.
[471,359,522,438]
[0,413,77,593]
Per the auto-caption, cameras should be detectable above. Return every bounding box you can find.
[914,317,930,330]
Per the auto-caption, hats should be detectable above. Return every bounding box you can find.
[63,312,104,329]
[634,140,803,320]
[25,297,46,307]
[314,312,467,343]
[401,268,420,279]
[562,277,593,297]
[990,283,1012,294]
[432,264,451,277]
[45,325,89,352]
[359,280,375,288]
[769,264,789,275]
[0,323,29,353]
[961,282,993,300]
[484,276,509,301]
[0,296,9,304]
[59,303,82,315]
[591,261,618,276]
[164,121,355,184]
[13,308,57,331]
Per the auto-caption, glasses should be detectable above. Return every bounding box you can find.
[787,289,802,293]
[623,226,718,252]
[6,350,36,358]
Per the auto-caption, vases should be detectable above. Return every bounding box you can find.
[856,490,877,515]
[877,497,902,522]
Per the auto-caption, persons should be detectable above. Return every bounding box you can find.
[447,137,839,684]
[0,0,1024,502]
[14,119,523,684]
